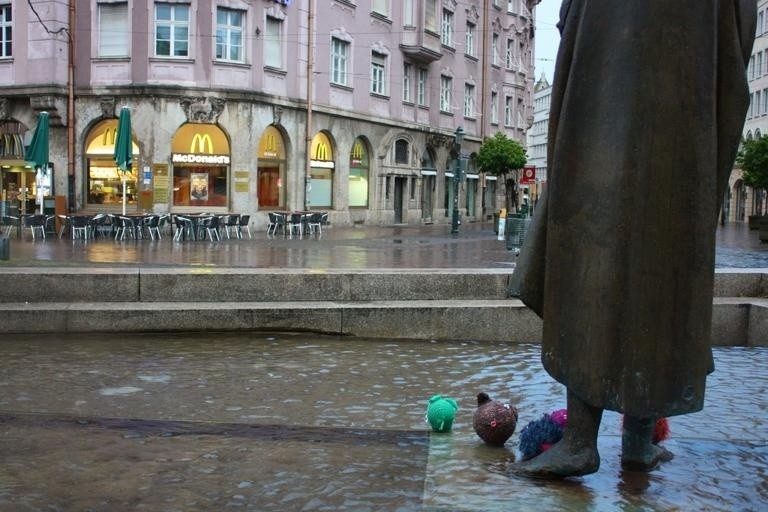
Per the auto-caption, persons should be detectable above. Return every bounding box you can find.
[505,1,756,477]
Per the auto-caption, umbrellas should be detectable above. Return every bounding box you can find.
[114,109,134,216]
[25,112,49,217]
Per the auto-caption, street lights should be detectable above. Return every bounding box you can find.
[451,124,466,235]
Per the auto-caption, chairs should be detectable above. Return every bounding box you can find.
[1,205,328,242]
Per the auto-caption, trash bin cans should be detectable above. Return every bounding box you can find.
[0,235,10,260]
[505,214,527,250]
[42,196,65,235]
[494,212,500,235]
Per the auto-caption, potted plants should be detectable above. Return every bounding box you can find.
[760,134,767,241]
[737,135,761,230]
[474,132,530,239]
